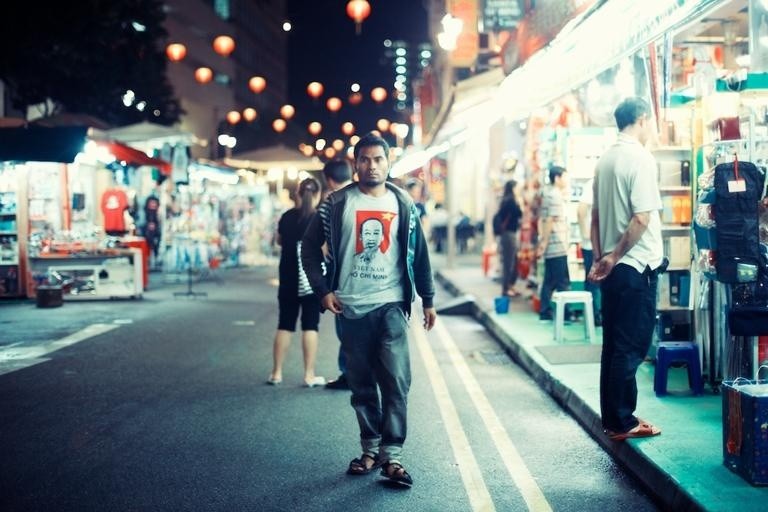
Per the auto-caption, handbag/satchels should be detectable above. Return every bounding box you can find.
[494,214,507,234]
[297,240,329,299]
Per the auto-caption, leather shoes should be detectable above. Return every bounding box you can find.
[328,379,344,388]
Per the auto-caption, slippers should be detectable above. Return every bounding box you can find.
[303,376,326,387]
[268,374,281,385]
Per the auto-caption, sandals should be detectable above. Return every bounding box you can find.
[347,451,380,475]
[379,460,413,487]
[609,423,661,442]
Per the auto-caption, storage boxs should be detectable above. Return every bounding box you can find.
[722,379,768,487]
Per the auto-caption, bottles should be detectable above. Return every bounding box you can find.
[31,234,39,257]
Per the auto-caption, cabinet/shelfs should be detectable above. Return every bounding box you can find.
[654,145,694,315]
[0,191,28,299]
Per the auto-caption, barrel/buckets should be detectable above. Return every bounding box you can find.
[494,296,511,314]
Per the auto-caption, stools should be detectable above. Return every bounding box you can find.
[553,291,595,344]
[655,342,704,398]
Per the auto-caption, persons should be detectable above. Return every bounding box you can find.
[323,159,354,389]
[352,218,385,273]
[301,134,436,486]
[266,178,327,387]
[588,98,663,441]
[395,167,602,325]
